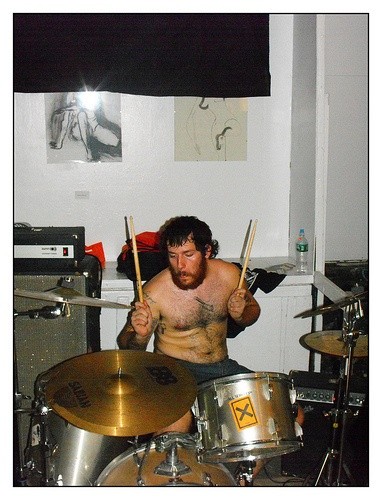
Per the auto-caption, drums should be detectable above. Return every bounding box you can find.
[24,375,133,487]
[90,435,235,488]
[197,370,299,462]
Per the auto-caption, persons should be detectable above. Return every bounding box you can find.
[116,216,305,487]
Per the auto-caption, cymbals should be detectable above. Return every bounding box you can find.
[34,347,198,436]
[13,284,134,312]
[296,330,369,357]
[294,291,369,317]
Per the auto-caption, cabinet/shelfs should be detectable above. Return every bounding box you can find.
[101,285,324,375]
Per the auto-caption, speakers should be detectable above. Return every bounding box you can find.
[282,369,369,483]
[13,253,100,478]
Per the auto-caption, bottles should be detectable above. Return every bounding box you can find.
[295,229,308,274]
